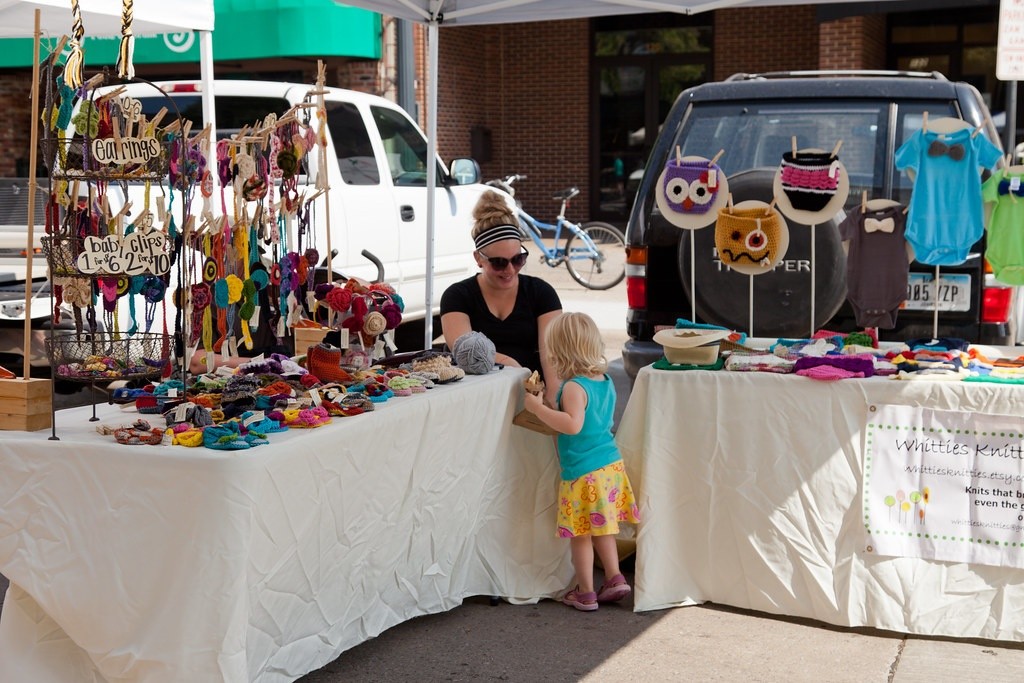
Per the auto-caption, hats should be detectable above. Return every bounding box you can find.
[781,150,841,212]
[714,206,781,269]
[651,328,733,366]
[665,160,720,215]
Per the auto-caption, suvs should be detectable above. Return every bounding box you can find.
[620,70,1019,394]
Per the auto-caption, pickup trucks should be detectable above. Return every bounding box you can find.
[0,81,522,367]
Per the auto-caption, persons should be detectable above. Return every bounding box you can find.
[525,312,642,611]
[188,242,291,376]
[440,191,564,411]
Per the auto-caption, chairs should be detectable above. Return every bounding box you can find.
[761,133,807,168]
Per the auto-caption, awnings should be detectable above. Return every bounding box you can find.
[0,0,386,69]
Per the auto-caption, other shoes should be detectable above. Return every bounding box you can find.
[114,342,466,451]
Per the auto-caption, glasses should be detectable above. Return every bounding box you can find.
[480,244,530,271]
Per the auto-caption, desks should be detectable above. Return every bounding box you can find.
[0,366,575,683]
[614,363,1024,645]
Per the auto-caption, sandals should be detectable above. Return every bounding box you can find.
[598,573,633,603]
[561,585,600,612]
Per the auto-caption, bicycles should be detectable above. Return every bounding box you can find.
[483,174,626,291]
